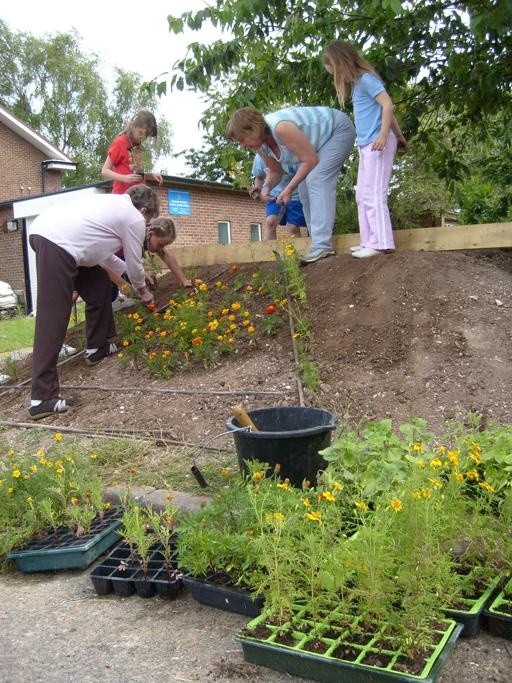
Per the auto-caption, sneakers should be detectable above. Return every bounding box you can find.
[84,342,124,365]
[112,296,136,312]
[350,245,364,251]
[352,246,386,258]
[27,396,80,420]
[301,249,336,262]
[59,343,77,358]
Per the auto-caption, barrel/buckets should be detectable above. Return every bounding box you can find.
[225,405,336,488]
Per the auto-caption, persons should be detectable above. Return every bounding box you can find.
[226,104,356,264]
[57,216,193,359]
[321,40,410,259]
[100,109,163,196]
[250,149,310,242]
[25,182,157,422]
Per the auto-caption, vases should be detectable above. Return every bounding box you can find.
[90,527,190,602]
[483,575,512,638]
[233,586,465,683]
[318,529,511,638]
[8,503,146,570]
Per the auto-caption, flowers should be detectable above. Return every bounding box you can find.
[116,487,185,564]
[0,431,110,556]
[317,407,512,602]
[244,462,464,661]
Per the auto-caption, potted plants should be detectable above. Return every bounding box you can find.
[175,463,293,616]
